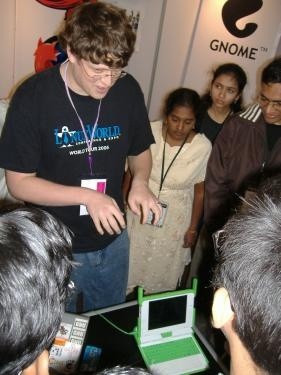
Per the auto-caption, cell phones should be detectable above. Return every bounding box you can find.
[79,343,102,375]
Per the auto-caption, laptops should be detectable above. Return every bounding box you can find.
[132,278,209,375]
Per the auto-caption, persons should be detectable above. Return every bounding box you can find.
[122,58,281,295]
[211,191,281,375]
[0,207,75,375]
[0,2,164,315]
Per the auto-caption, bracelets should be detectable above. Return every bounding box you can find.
[189,230,198,234]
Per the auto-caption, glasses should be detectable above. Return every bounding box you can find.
[78,54,128,82]
